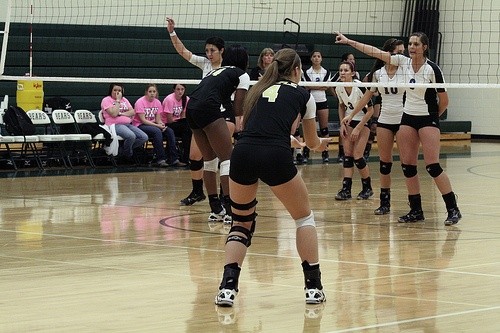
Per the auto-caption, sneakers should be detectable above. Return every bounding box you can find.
[374,204,390,215]
[179,190,205,206]
[218,199,232,216]
[444,207,461,225]
[302,285,326,303]
[334,187,351,200]
[356,188,374,200]
[296,155,302,164]
[215,286,239,307]
[364,154,369,162]
[208,205,232,224]
[322,155,329,164]
[301,150,310,163]
[398,209,424,223]
[337,154,344,162]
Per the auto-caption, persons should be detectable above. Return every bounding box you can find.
[101,83,149,174]
[332,60,375,201]
[299,50,340,165]
[165,16,236,208]
[358,54,392,163]
[340,38,421,224]
[333,28,462,226]
[213,49,334,307]
[248,47,280,94]
[184,46,253,224]
[132,82,186,170]
[161,79,198,168]
[327,51,361,163]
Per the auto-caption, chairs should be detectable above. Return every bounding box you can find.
[0,110,45,173]
[52,108,94,169]
[98,109,141,168]
[26,109,66,173]
[75,108,109,169]
